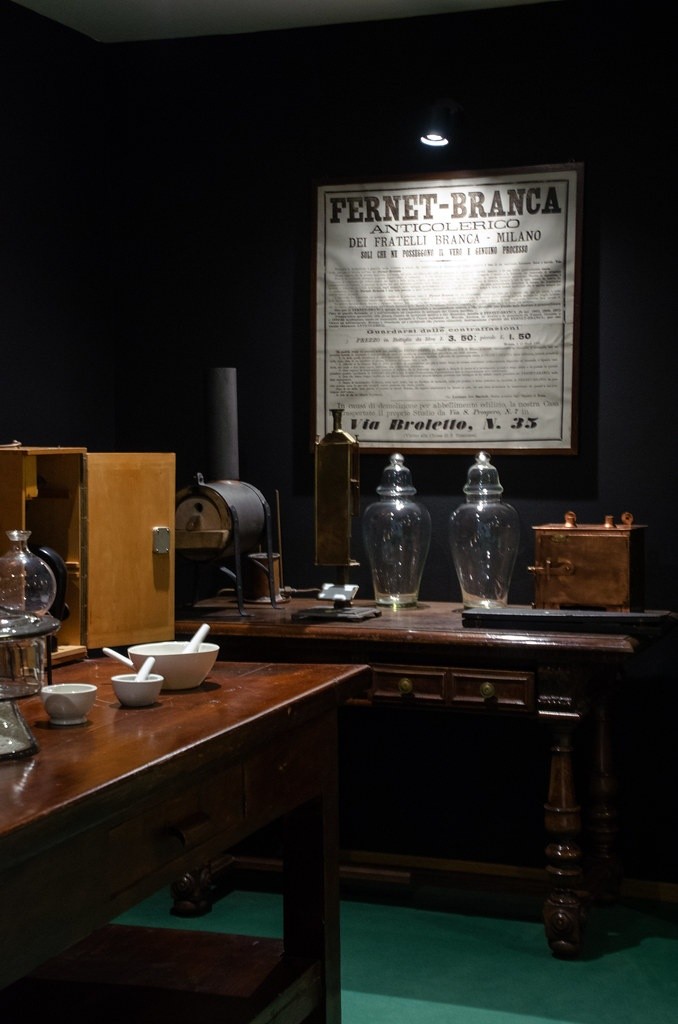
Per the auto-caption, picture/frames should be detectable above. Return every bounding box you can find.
[304,161,589,467]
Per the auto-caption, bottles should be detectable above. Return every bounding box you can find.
[445,451,522,611]
[362,453,433,610]
[0,530,58,622]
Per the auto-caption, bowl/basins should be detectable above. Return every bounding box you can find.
[112,674,165,706]
[127,641,220,690]
[40,683,98,724]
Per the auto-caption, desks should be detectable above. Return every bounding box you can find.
[0,651,372,1024]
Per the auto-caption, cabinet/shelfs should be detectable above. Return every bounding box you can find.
[0,439,177,679]
[173,579,676,959]
